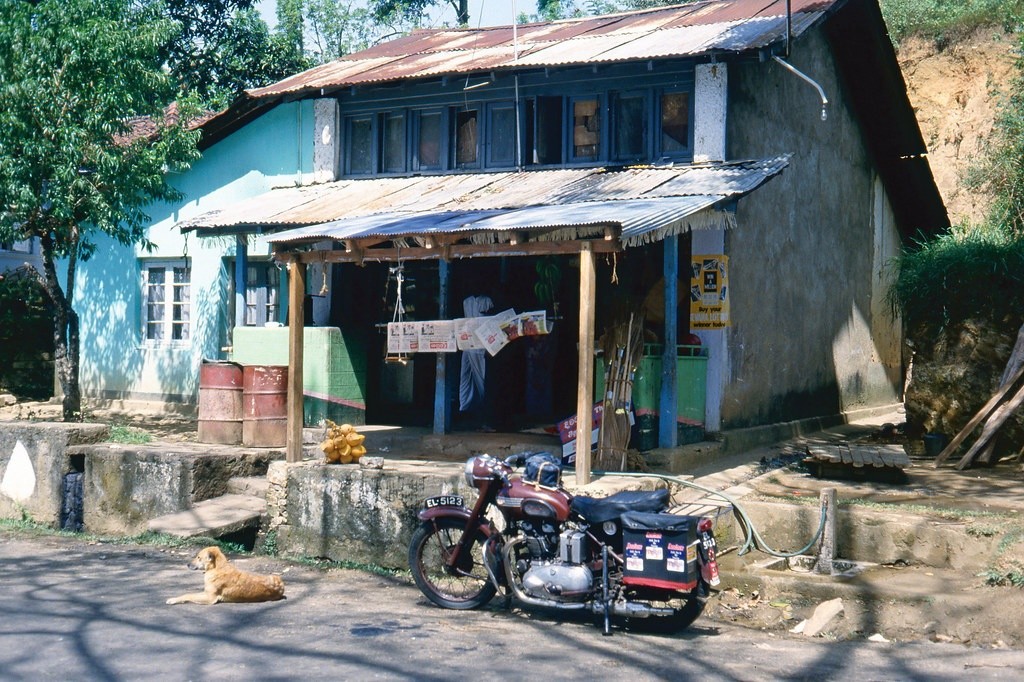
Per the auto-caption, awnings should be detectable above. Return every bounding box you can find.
[178,155,792,492]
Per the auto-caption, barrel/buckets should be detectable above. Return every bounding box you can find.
[242,365,288,448]
[196,363,243,445]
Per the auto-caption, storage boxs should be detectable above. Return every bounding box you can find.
[197,358,289,447]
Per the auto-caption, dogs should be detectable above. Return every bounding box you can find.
[166,547,285,605]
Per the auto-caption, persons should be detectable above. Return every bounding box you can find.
[454,274,499,434]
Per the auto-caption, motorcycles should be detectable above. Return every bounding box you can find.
[410,450,722,638]
